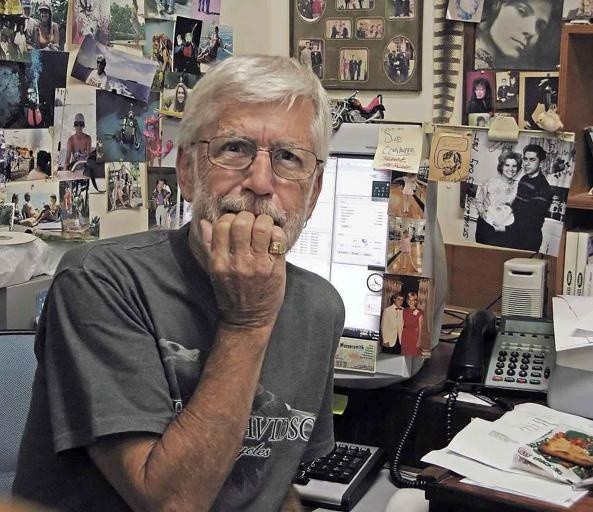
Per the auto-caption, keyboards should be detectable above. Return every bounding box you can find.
[291,441,385,512]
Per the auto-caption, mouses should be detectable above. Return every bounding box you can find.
[385,488,430,512]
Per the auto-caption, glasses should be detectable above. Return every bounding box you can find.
[191,137,323,182]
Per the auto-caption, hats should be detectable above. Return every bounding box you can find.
[39,5,48,10]
[73,113,84,123]
[21,1,31,10]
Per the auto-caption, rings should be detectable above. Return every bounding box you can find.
[270,241,291,255]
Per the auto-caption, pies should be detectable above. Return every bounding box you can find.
[540,435,592,468]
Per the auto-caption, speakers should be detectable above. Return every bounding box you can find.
[501,258,548,319]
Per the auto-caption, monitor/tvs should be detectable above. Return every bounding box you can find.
[285,123,447,389]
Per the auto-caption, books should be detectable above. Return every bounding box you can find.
[574,229,593,297]
[561,225,588,297]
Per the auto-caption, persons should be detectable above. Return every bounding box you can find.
[465,76,493,112]
[399,291,423,354]
[506,75,519,104]
[399,230,419,272]
[476,0,559,70]
[379,291,404,355]
[1,1,237,239]
[474,149,524,246]
[392,172,425,212]
[494,143,554,249]
[497,78,510,103]
[14,53,345,511]
[296,1,420,88]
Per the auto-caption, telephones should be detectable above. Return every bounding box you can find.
[447,310,555,398]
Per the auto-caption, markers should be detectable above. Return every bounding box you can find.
[471,386,516,411]
